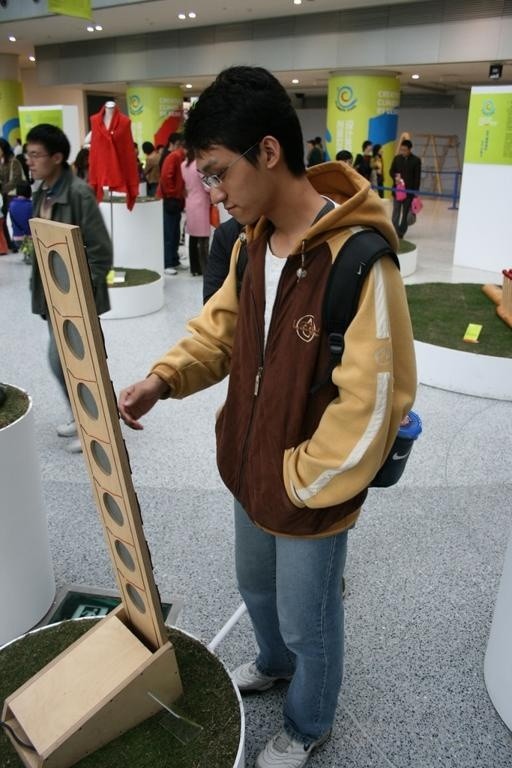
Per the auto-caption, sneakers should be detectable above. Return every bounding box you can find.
[66,440,82,454]
[230,662,276,694]
[165,267,177,275]
[254,723,331,767]
[177,263,188,270]
[55,421,76,437]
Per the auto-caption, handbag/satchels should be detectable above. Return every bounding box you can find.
[209,205,220,228]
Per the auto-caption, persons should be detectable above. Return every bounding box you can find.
[307,136,324,161]
[336,150,353,166]
[370,144,385,198]
[0,130,234,276]
[116,64,417,767]
[390,139,422,238]
[306,139,323,167]
[24,121,114,453]
[353,141,372,181]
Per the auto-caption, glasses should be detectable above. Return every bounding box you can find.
[24,152,50,160]
[200,148,254,189]
